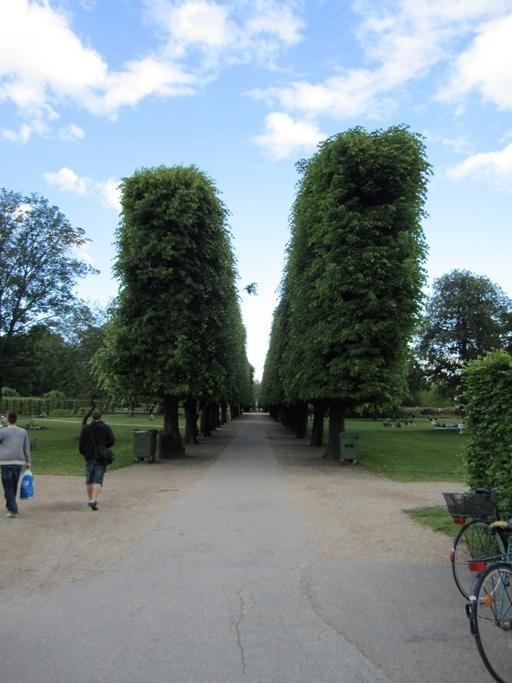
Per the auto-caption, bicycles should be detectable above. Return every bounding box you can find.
[440,477,512,683]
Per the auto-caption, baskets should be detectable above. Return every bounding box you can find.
[442,492,497,515]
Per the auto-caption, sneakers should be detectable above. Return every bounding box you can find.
[4,502,17,518]
[87,499,98,511]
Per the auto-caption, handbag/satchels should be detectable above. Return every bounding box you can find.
[94,445,113,465]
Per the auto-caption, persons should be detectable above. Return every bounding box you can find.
[0,411,31,518]
[78,409,116,510]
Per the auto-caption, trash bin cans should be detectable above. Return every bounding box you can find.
[131,429,159,464]
[338,431,360,465]
